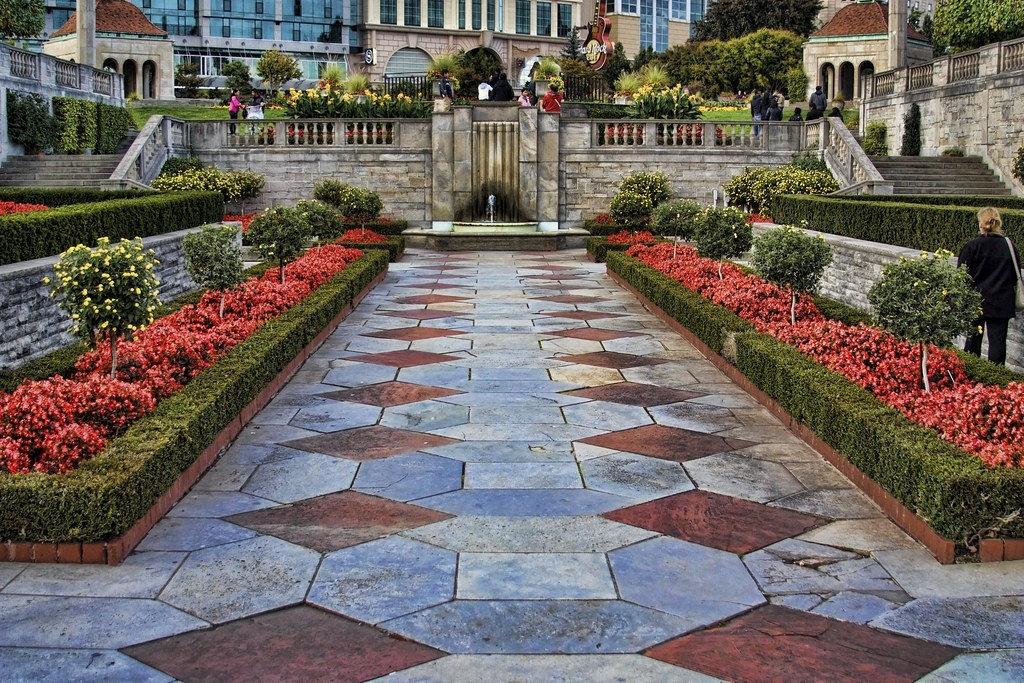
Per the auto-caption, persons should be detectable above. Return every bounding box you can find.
[542,84,561,112]
[809,86,827,112]
[478,73,514,101]
[517,87,537,106]
[805,102,823,122]
[750,89,784,136]
[248,88,266,134]
[439,68,452,100]
[957,206,1021,368]
[788,107,804,121]
[228,88,245,134]
[828,107,844,122]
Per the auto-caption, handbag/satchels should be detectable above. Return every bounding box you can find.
[1015,279,1024,309]
